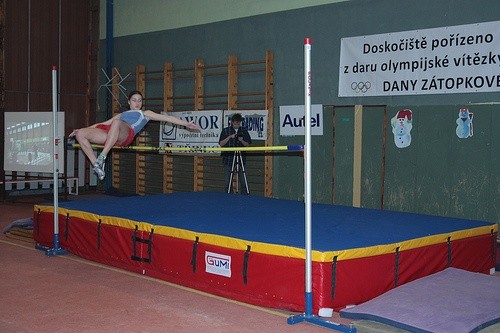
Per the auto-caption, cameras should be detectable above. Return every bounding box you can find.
[234,133,241,140]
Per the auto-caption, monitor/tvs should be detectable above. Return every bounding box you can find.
[4,112,65,174]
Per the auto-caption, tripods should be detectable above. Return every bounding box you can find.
[227,140,250,194]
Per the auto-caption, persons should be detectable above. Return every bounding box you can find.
[219,114,253,196]
[68,91,199,181]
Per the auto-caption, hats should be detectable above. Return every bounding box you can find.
[232,114,244,122]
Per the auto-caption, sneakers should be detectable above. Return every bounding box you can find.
[94,154,106,179]
